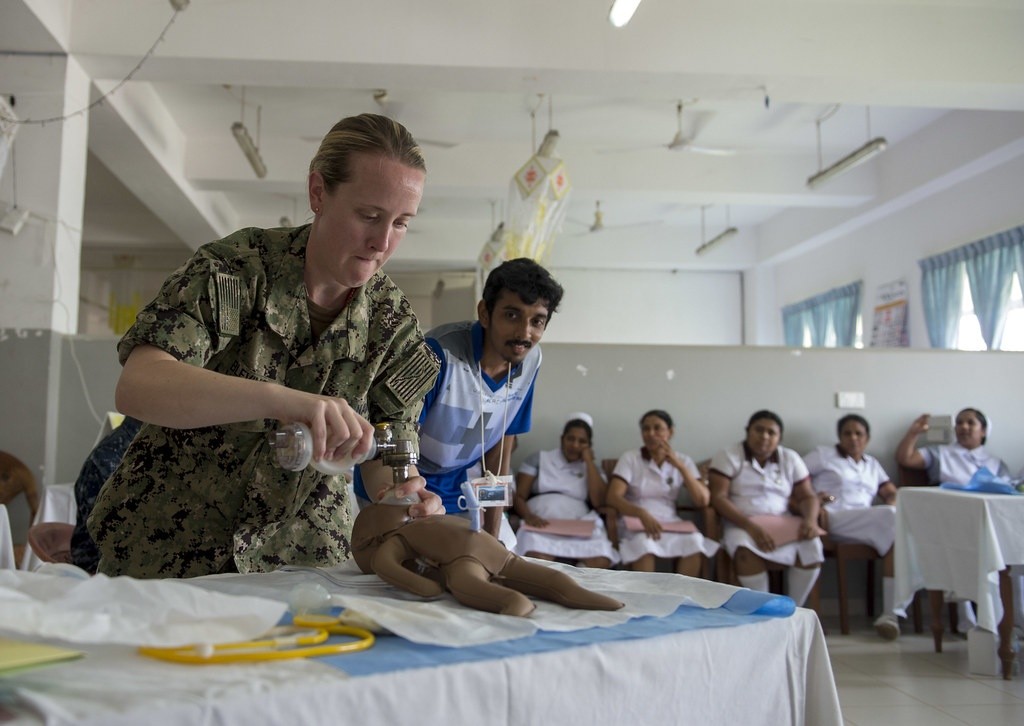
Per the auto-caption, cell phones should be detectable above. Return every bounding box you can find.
[926,417,952,445]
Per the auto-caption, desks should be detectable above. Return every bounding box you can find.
[0,557,844,726]
[892,485,1024,680]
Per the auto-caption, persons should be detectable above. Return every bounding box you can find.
[802,414,900,638]
[896,408,1018,631]
[350,502,624,617]
[353,257,564,540]
[606,409,720,578]
[68,415,146,578]
[513,412,621,569]
[709,409,828,607]
[85,113,446,580]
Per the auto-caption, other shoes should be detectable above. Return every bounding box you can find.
[874,615,900,640]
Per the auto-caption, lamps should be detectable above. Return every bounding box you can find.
[696,203,739,256]
[230,86,268,179]
[806,104,890,191]
[529,94,560,158]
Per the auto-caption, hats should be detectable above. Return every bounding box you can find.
[567,413,593,428]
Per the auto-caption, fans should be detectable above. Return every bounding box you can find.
[595,100,734,157]
[565,202,663,237]
[297,92,460,149]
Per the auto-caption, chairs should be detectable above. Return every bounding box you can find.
[600,458,959,635]
